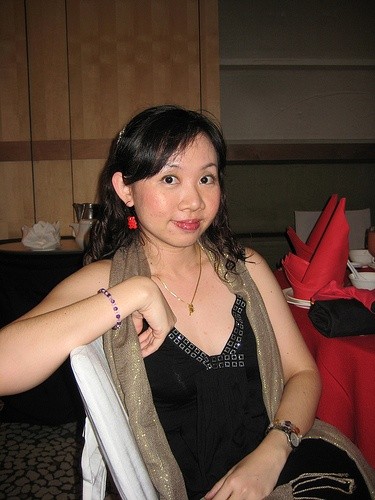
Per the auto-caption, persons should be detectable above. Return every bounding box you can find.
[0,104,375,500]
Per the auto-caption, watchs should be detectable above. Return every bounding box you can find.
[268,425,301,448]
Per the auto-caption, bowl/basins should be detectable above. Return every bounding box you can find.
[350,247,373,268]
[348,272,375,291]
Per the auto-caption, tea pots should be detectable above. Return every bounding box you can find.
[68,220,100,252]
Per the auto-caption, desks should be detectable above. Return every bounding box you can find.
[273,266,375,469]
[0,235,79,424]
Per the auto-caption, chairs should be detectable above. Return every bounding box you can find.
[295,209,371,249]
[70,334,159,500]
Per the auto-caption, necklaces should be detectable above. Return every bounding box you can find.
[148,245,204,313]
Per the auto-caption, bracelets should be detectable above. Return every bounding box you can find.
[272,421,303,438]
[96,288,121,331]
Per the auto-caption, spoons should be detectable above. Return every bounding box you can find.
[344,260,366,279]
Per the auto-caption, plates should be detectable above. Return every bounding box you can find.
[287,289,313,303]
[281,287,314,309]
